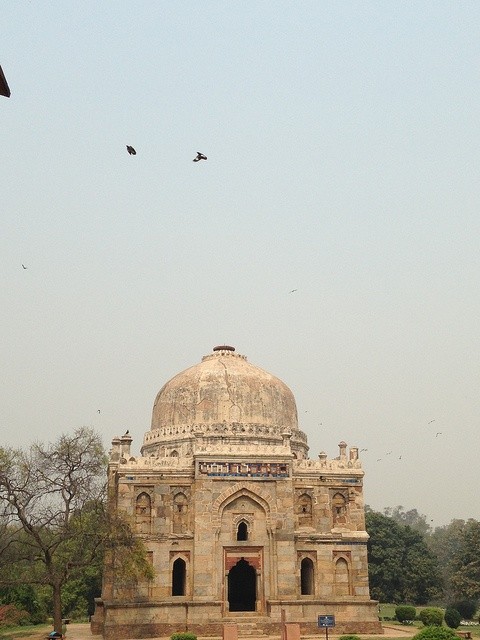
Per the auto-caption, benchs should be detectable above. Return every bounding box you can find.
[61,619,71,624]
[45,635,66,640]
[456,630,472,638]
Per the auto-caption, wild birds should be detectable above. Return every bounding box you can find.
[193,152,207,162]
[126,145,136,155]
